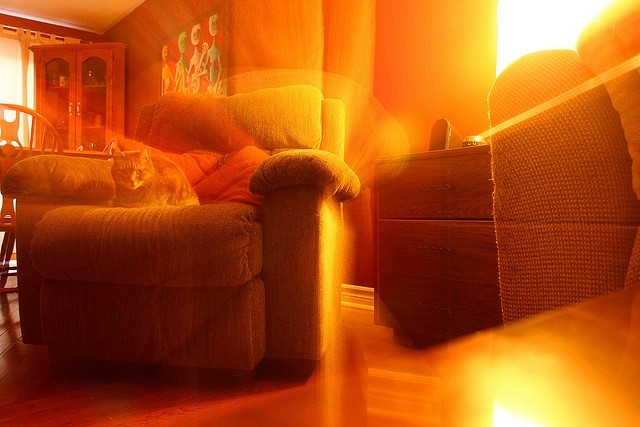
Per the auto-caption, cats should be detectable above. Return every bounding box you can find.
[110,146,200,208]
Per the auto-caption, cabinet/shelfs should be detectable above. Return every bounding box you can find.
[370,144,504,350]
[28,42,127,152]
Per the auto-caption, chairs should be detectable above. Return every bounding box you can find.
[0,103,66,293]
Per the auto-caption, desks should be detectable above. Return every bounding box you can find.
[1,146,113,276]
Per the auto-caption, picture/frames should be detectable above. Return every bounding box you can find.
[158,1,233,97]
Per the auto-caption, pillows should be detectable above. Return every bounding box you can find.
[117,139,226,187]
[191,145,271,207]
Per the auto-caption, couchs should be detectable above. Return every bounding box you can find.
[488,0,639,326]
[0,84,362,380]
